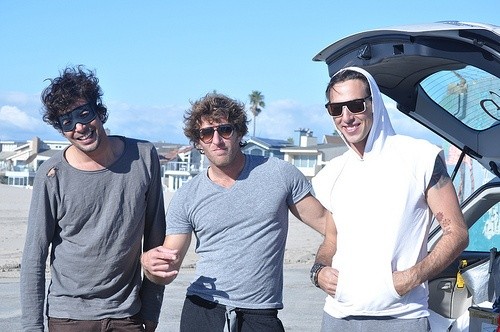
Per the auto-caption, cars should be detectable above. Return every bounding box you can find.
[312,21,500,332]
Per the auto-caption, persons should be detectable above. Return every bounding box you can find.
[20,64,167,332]
[309,67,469,332]
[140,90,327,332]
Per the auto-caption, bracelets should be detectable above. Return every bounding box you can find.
[310,262,326,287]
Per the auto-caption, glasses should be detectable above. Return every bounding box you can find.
[324,95,372,117]
[198,124,238,144]
